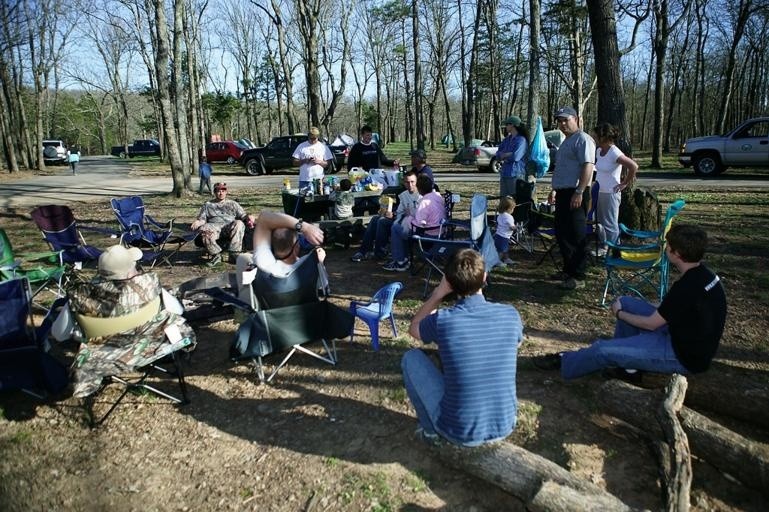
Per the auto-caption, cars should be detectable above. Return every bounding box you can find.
[197,140,248,163]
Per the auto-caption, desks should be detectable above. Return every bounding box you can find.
[282,186,404,225]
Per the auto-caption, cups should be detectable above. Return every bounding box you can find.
[283,179,291,190]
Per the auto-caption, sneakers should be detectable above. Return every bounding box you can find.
[412,425,447,448]
[530,351,567,371]
[229,252,241,264]
[604,367,645,388]
[375,255,388,265]
[383,261,407,272]
[552,270,571,281]
[559,277,586,291]
[351,251,370,263]
[591,248,610,258]
[404,257,412,268]
[205,254,223,267]
[504,259,513,264]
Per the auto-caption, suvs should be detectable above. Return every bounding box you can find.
[42,139,68,166]
[674,116,769,179]
[236,133,349,176]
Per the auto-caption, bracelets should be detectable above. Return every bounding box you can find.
[576,188,583,194]
[616,308,622,319]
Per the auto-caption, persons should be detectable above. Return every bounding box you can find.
[291,124,448,272]
[400,247,525,446]
[589,122,639,257]
[236,211,327,312]
[495,116,529,202]
[67,141,81,176]
[546,106,597,290]
[190,183,254,267]
[531,223,727,380]
[51,244,184,342]
[196,156,213,195]
[493,195,519,265]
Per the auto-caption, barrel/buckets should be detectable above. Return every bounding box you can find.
[348,165,368,187]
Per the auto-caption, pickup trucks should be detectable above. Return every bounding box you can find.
[110,138,161,157]
[470,133,563,175]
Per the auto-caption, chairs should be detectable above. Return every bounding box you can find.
[229,246,339,386]
[32,204,106,277]
[0,227,70,312]
[67,272,198,430]
[1,277,75,423]
[600,199,686,306]
[349,282,403,350]
[109,194,202,273]
[400,188,500,300]
[497,177,564,273]
[193,221,254,264]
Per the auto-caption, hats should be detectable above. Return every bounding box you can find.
[554,106,577,122]
[409,149,427,159]
[213,182,227,192]
[501,116,522,127]
[97,244,144,281]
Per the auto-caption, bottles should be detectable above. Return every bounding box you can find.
[310,177,338,195]
[247,213,254,229]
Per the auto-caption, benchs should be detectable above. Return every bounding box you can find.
[313,215,404,249]
